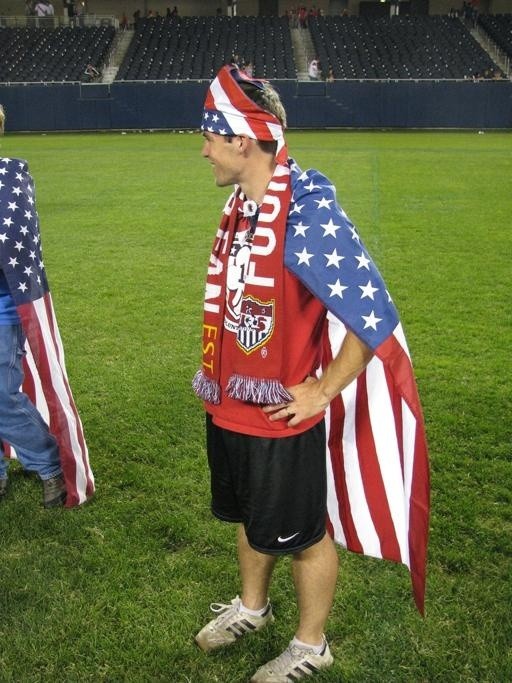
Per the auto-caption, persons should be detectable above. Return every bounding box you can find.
[307,57,323,80]
[491,69,503,79]
[23,0,179,31]
[84,62,103,82]
[328,64,334,79]
[467,71,484,80]
[193,62,375,682]
[284,5,348,31]
[0,100,68,510]
[462,1,475,33]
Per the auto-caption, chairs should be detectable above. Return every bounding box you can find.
[0,11,512,82]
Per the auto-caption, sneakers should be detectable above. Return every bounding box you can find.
[249,633,334,682]
[195,597,276,652]
